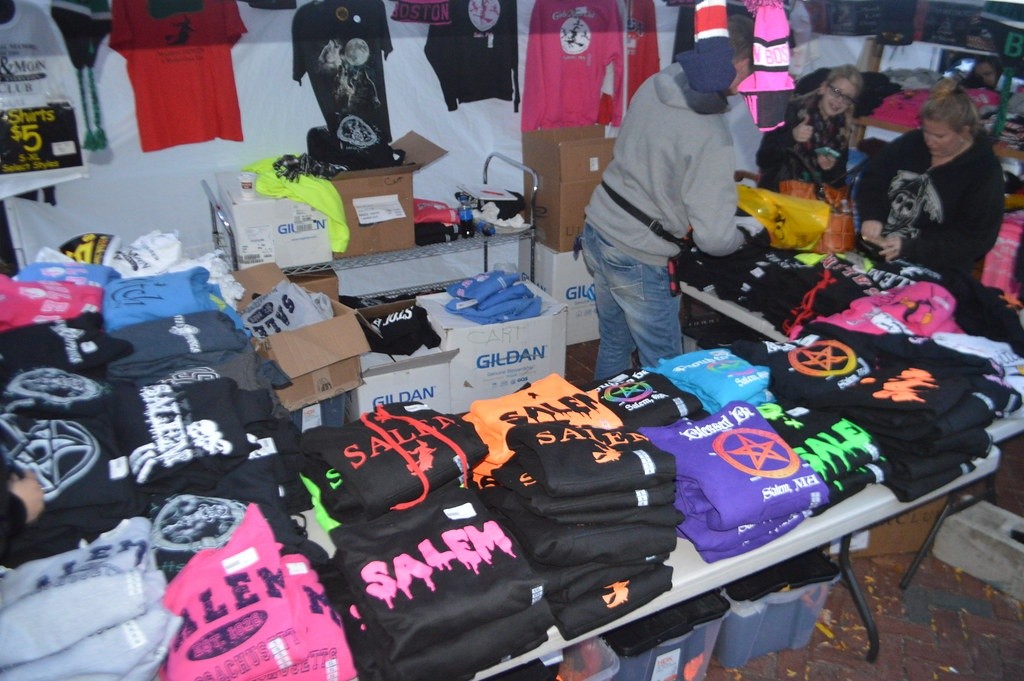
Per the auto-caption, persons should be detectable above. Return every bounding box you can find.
[0,450,47,563]
[974,56,1004,88]
[860,78,1006,286]
[582,11,765,383]
[755,65,864,191]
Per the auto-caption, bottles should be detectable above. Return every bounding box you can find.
[459,196,475,238]
[840,200,852,213]
[475,218,495,237]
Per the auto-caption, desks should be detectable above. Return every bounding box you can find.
[463,280,1024,681]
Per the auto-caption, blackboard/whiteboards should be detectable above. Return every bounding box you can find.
[827,1,916,46]
[919,1,985,49]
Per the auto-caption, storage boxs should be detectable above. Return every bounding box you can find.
[519,240,601,346]
[713,567,844,667]
[217,158,336,267]
[616,589,731,681]
[329,131,450,260]
[552,632,620,681]
[414,274,567,412]
[227,268,464,431]
[823,495,948,559]
[521,124,617,251]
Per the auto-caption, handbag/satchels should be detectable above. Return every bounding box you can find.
[737,184,831,250]
[779,148,861,253]
[601,179,744,282]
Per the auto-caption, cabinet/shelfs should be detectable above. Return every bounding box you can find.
[202,153,540,306]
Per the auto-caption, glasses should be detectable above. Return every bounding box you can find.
[827,84,855,105]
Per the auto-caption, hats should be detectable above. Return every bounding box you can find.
[50,0,113,152]
